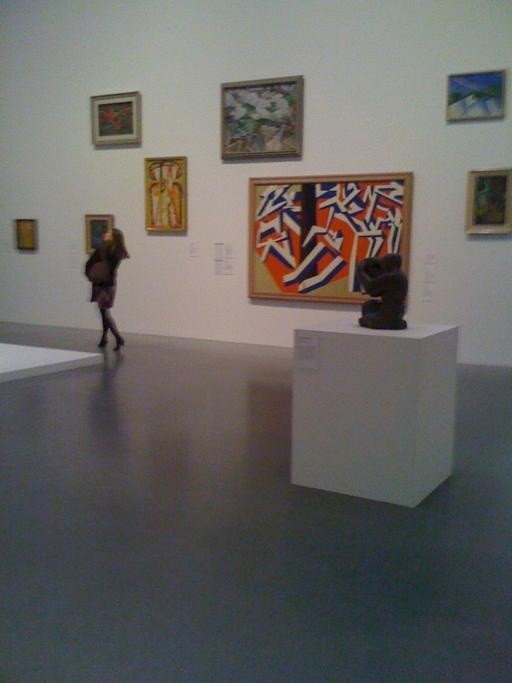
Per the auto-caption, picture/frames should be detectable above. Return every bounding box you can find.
[464,169,511,235]
[143,156,187,233]
[15,219,36,250]
[247,171,414,304]
[222,75,304,159]
[90,91,141,146]
[445,69,505,122]
[85,214,114,254]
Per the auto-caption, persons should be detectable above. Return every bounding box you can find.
[84,227,130,350]
[355,251,410,331]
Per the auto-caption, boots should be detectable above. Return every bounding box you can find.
[107,319,125,351]
[98,317,109,347]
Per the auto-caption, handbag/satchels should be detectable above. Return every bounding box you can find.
[88,260,112,284]
